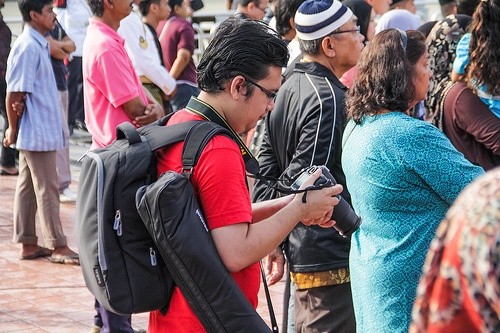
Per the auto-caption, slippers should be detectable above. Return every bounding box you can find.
[51,251,80,265]
[20,247,53,260]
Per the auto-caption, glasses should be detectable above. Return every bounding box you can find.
[257,6,269,13]
[330,26,361,34]
[231,71,278,102]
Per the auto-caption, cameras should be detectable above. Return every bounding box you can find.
[290,165,362,237]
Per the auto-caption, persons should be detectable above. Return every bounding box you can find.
[0,0,500,204]
[148,14,343,333]
[81,0,146,333]
[409,167,500,333]
[252,0,364,333]
[341,29,485,333]
[3,0,81,265]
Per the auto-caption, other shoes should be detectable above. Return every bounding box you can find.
[0,170,18,176]
[134,328,145,332]
[90,327,99,333]
[60,189,77,203]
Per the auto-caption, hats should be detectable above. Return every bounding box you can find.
[295,0,353,40]
[373,7,420,37]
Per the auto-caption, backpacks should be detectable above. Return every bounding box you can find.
[76,109,233,315]
